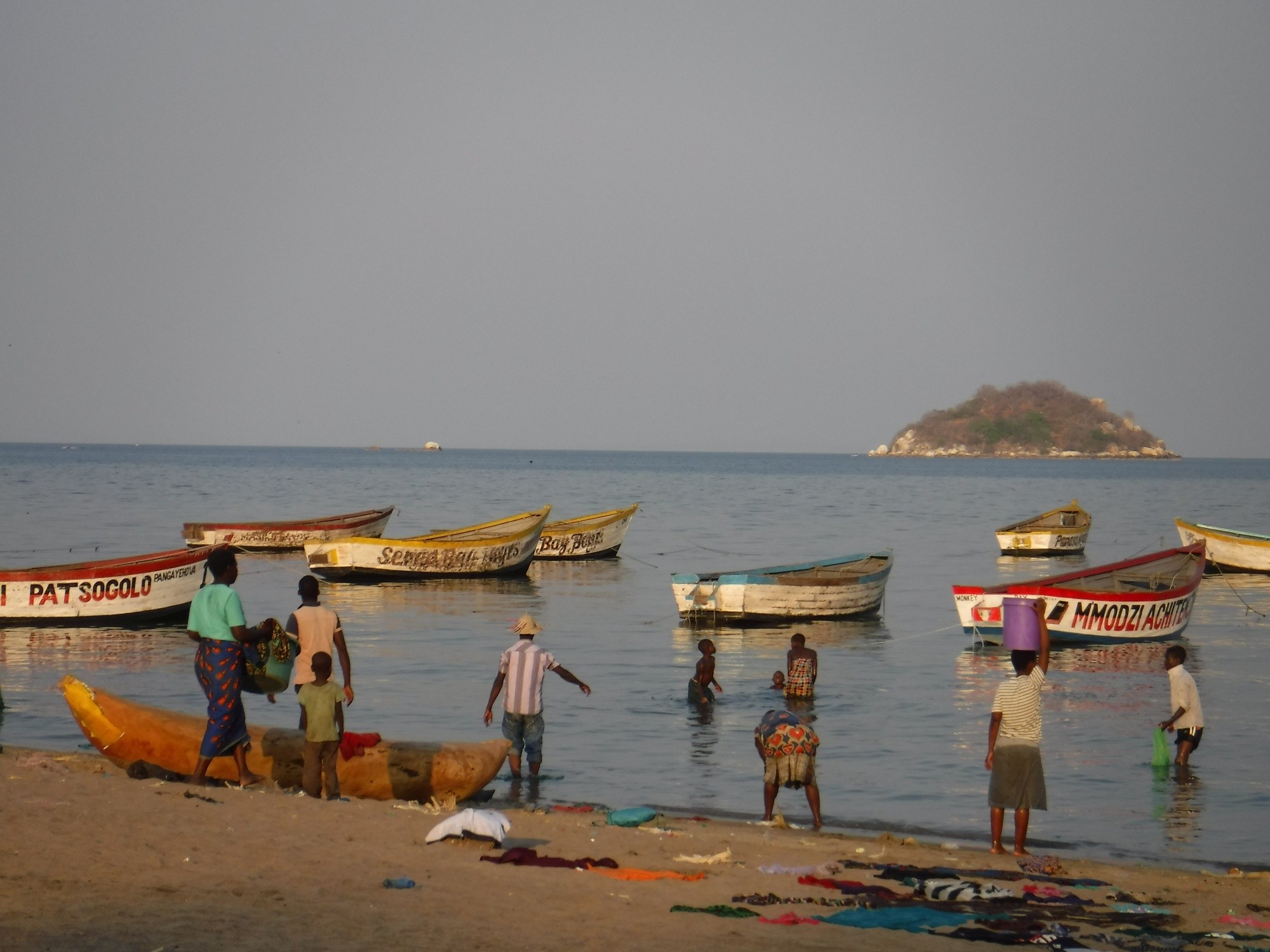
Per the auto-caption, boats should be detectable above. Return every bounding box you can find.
[429,503,639,560]
[1173,516,1270,572]
[0,534,234,627]
[951,537,1207,648]
[54,672,515,806]
[183,506,395,552]
[670,546,894,623]
[302,505,554,577]
[995,500,1094,556]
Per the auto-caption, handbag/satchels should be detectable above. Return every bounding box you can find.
[1152,728,1170,767]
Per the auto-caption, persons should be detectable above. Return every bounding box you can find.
[688,639,722,704]
[754,709,822,830]
[482,614,591,776]
[770,671,786,689]
[185,547,273,787]
[783,633,817,699]
[983,597,1049,858]
[267,575,355,732]
[297,651,350,801]
[1157,645,1204,768]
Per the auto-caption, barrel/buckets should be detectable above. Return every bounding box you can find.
[240,635,301,695]
[1002,597,1040,651]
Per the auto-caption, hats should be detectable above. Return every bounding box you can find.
[507,613,543,635]
